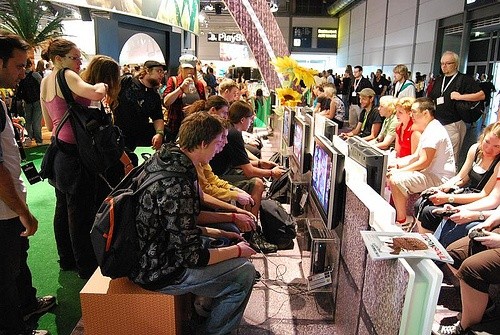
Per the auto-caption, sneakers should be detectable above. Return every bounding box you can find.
[396,216,413,232]
[432,321,470,335]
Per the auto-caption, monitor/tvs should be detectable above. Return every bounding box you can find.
[281,104,389,230]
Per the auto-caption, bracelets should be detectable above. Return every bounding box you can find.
[376,138,379,142]
[232,213,236,223]
[236,244,241,258]
[179,86,184,91]
[396,164,399,169]
[271,170,273,176]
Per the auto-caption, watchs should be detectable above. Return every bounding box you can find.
[156,129,164,136]
[479,212,485,221]
[258,161,261,168]
[448,194,454,203]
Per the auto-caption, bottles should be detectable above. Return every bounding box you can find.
[187,74,197,94]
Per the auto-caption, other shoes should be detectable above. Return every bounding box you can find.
[242,232,278,253]
[21,295,56,322]
[28,329,49,335]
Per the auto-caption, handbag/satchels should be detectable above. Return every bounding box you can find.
[454,89,485,123]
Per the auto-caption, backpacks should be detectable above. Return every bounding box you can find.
[89,164,187,279]
[265,169,290,202]
[259,199,296,242]
[20,71,40,104]
[56,67,125,168]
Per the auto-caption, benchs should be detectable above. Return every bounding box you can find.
[79,265,190,335]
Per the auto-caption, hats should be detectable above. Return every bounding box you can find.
[144,60,167,70]
[179,54,198,68]
[358,88,375,97]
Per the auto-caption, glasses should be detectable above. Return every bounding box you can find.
[158,70,164,74]
[69,56,83,61]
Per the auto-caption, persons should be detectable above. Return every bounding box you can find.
[89,110,257,335]
[0,30,500,335]
